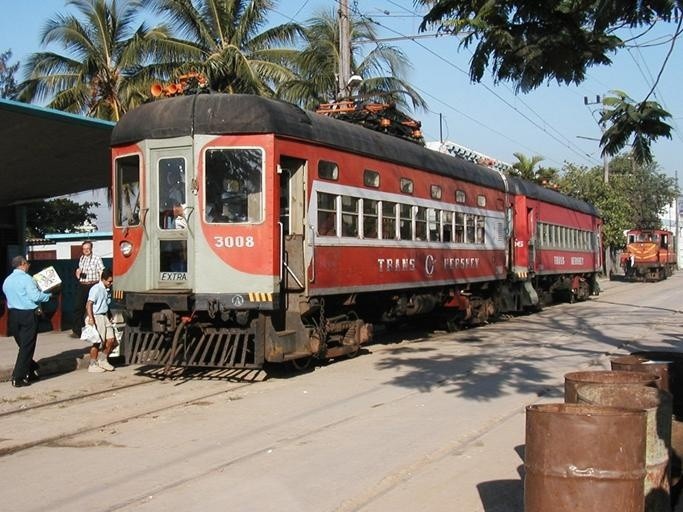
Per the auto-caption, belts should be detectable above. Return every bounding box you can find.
[8,309,23,312]
[94,313,106,315]
[80,281,97,285]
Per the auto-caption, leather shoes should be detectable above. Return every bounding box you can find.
[12,377,32,387]
[26,366,40,381]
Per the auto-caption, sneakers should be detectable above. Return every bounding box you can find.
[98,359,114,371]
[88,365,105,373]
[69,331,81,338]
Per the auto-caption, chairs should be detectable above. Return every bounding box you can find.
[217,178,240,217]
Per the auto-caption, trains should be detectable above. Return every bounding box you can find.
[108,71,604,376]
[620,227,676,282]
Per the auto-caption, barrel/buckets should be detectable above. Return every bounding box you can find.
[522,346,682,512]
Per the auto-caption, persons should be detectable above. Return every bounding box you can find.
[67,241,105,338]
[162,198,187,229]
[84,268,117,374]
[2,256,53,387]
